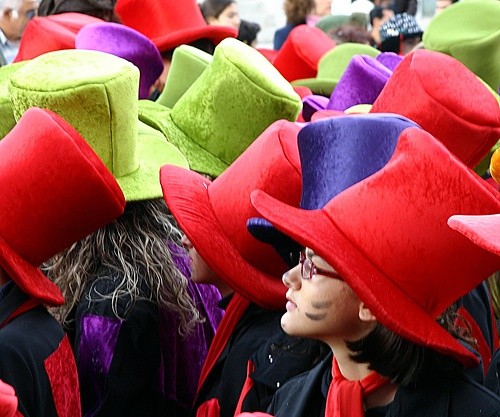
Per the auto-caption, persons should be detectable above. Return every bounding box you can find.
[0,0,500,417]
[249,128,500,417]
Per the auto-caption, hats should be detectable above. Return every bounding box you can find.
[422,0,500,95]
[257,26,336,83]
[138,37,302,178]
[155,43,214,110]
[0,48,191,201]
[380,13,424,42]
[0,105,126,306]
[251,127,500,366]
[302,54,393,112]
[75,22,163,100]
[114,0,238,54]
[291,43,382,98]
[368,48,500,171]
[311,104,373,123]
[159,118,299,311]
[246,114,417,269]
[13,12,107,66]
[376,51,405,74]
[447,214,500,256]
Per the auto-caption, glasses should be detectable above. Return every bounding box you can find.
[10,9,40,21]
[297,248,348,279]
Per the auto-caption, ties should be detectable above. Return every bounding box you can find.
[324,357,390,417]
